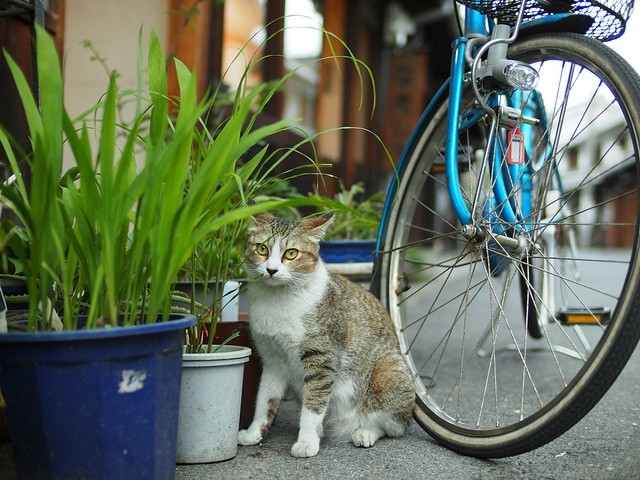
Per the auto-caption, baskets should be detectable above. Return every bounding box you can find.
[454,0,634,42]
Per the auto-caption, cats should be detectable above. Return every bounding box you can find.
[238,199,416,458]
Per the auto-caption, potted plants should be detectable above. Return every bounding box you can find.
[0,22,333,480]
[303,179,386,261]
[89,13,407,466]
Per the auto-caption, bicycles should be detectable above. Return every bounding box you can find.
[369,0,639,459]
